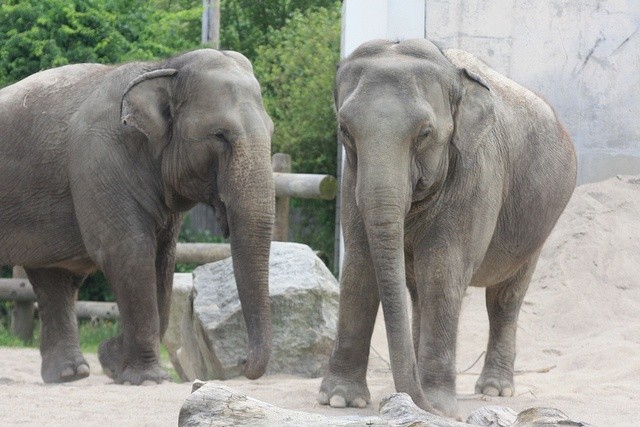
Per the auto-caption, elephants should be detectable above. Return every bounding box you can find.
[317,37,578,418]
[0,47,275,385]
